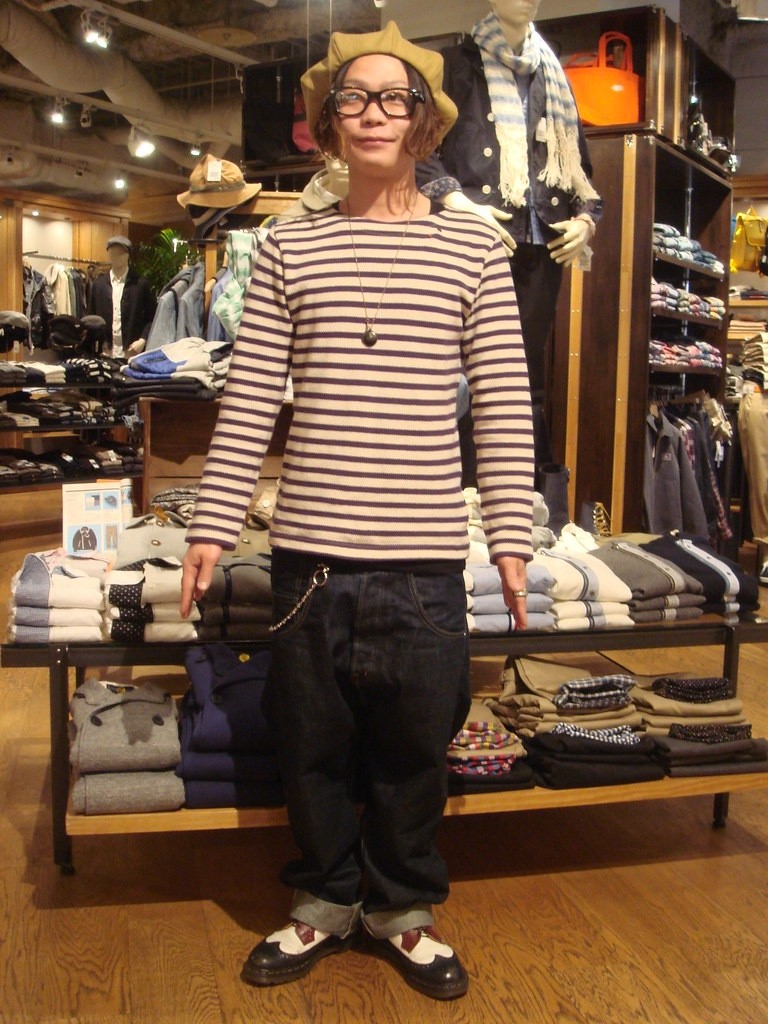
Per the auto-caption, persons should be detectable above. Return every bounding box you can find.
[179,21,534,997]
[89,235,156,363]
[415,0,603,492]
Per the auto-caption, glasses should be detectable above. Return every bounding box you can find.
[329,87,425,119]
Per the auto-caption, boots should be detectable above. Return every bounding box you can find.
[580,501,612,537]
[538,463,570,541]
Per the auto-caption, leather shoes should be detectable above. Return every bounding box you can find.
[357,916,469,1001]
[244,918,357,986]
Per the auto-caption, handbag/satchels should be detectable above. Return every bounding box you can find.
[293,79,321,153]
[242,57,317,163]
[561,30,646,128]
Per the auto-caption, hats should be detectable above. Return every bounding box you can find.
[300,20,458,157]
[80,315,106,330]
[177,154,262,242]
[47,314,85,335]
[106,236,133,253]
[0,311,30,329]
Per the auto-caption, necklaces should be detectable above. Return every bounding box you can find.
[346,191,418,347]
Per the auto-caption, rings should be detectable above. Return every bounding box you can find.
[513,589,527,597]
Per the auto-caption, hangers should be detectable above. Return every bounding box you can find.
[179,239,230,293]
[650,391,700,421]
[50,256,98,271]
[22,254,30,267]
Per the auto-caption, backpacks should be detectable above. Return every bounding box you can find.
[729,206,768,279]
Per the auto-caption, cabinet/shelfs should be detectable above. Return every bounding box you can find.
[0,379,145,496]
[0,619,767,877]
[531,133,732,536]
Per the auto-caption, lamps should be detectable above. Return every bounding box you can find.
[80,10,98,42]
[80,103,94,127]
[97,17,114,47]
[128,123,155,157]
